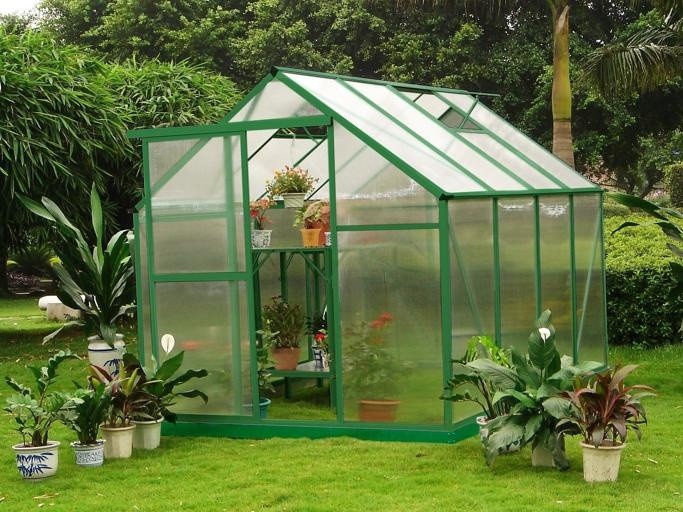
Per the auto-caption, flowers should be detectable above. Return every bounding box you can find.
[343,311,420,401]
[316,332,328,354]
[263,163,319,195]
[250,198,270,229]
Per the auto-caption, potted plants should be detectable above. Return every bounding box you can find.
[303,304,327,371]
[54,379,113,469]
[436,305,654,482]
[292,198,331,248]
[104,348,209,452]
[15,179,137,382]
[0,347,83,482]
[85,362,138,459]
[243,293,306,419]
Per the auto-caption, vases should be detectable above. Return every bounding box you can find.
[359,398,398,422]
[321,352,329,371]
[251,230,272,248]
[283,193,307,208]
